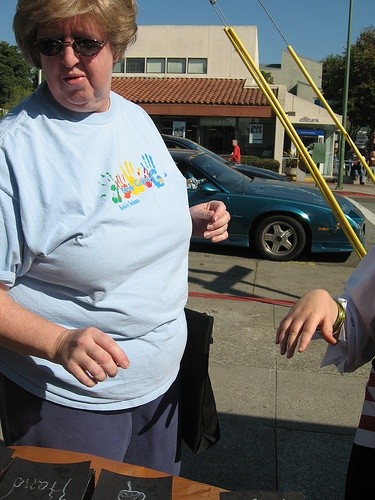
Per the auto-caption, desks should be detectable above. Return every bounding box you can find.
[6,445,231,500]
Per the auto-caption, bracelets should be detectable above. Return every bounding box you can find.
[315,298,345,335]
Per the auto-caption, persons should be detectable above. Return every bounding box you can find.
[184,169,216,186]
[0,0,232,476]
[275,243,375,500]
[344,133,375,184]
[224,139,241,164]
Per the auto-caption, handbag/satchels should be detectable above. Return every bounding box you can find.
[182,305,223,455]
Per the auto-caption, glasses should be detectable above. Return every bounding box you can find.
[29,35,112,56]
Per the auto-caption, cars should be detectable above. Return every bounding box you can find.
[159,132,292,182]
[167,147,368,261]
